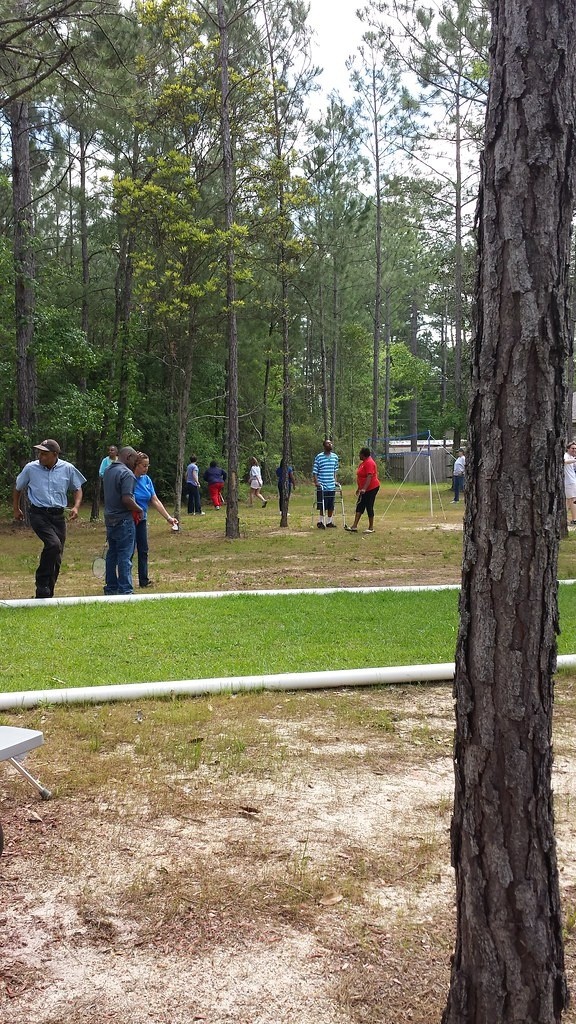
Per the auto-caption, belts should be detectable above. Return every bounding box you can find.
[31,505,64,514]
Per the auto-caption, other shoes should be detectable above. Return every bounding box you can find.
[571,520,576,524]
[215,506,219,511]
[317,522,325,529]
[363,529,374,533]
[262,501,267,508]
[450,500,459,504]
[326,522,336,527]
[189,512,195,516]
[246,503,253,508]
[196,511,206,516]
[345,527,358,533]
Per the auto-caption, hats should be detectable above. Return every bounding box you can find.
[455,448,464,452]
[34,439,61,453]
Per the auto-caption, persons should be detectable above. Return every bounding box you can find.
[202,460,227,510]
[276,459,296,515]
[246,457,268,508]
[99,446,178,596]
[563,442,576,526]
[184,457,206,516]
[345,447,380,533]
[14,439,87,599]
[312,440,338,528]
[449,448,466,504]
